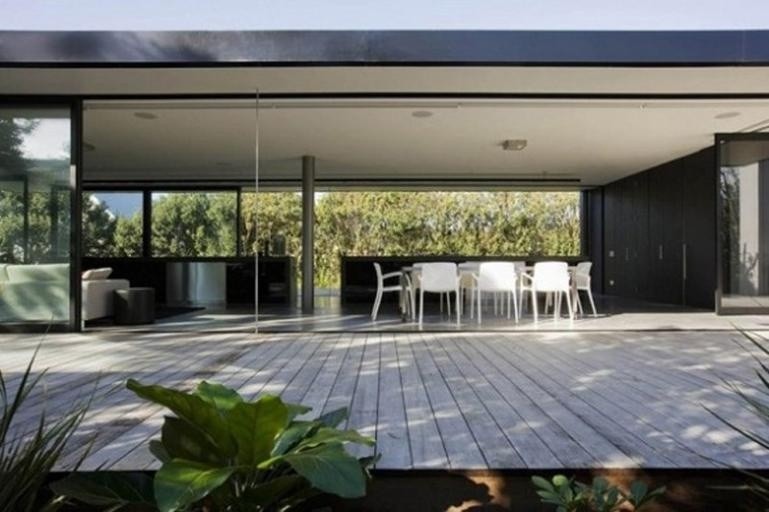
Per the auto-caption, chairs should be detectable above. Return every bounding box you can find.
[470,262,518,323]
[418,263,460,331]
[557,261,597,317]
[370,263,415,321]
[518,261,574,326]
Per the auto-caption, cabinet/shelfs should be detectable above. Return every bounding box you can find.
[90,257,300,311]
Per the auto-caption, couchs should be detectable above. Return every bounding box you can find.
[0,263,70,325]
[81,278,130,332]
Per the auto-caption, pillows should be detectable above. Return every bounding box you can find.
[82,267,112,278]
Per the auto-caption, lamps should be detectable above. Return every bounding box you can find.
[502,140,527,151]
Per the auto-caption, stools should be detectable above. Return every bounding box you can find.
[128,287,155,324]
[112,289,128,325]
